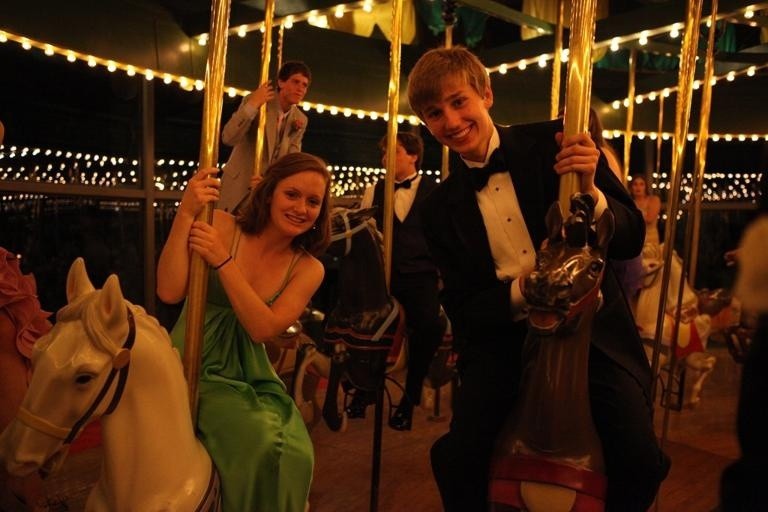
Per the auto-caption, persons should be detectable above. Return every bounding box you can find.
[409,46,674,510]
[555,103,623,186]
[212,61,327,326]
[345,130,444,430]
[154,151,333,511]
[1,200,767,512]
[629,175,663,260]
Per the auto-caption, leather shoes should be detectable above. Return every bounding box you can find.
[346,391,374,419]
[390,395,422,431]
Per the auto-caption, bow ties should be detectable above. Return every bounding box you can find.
[395,179,410,191]
[466,147,510,191]
